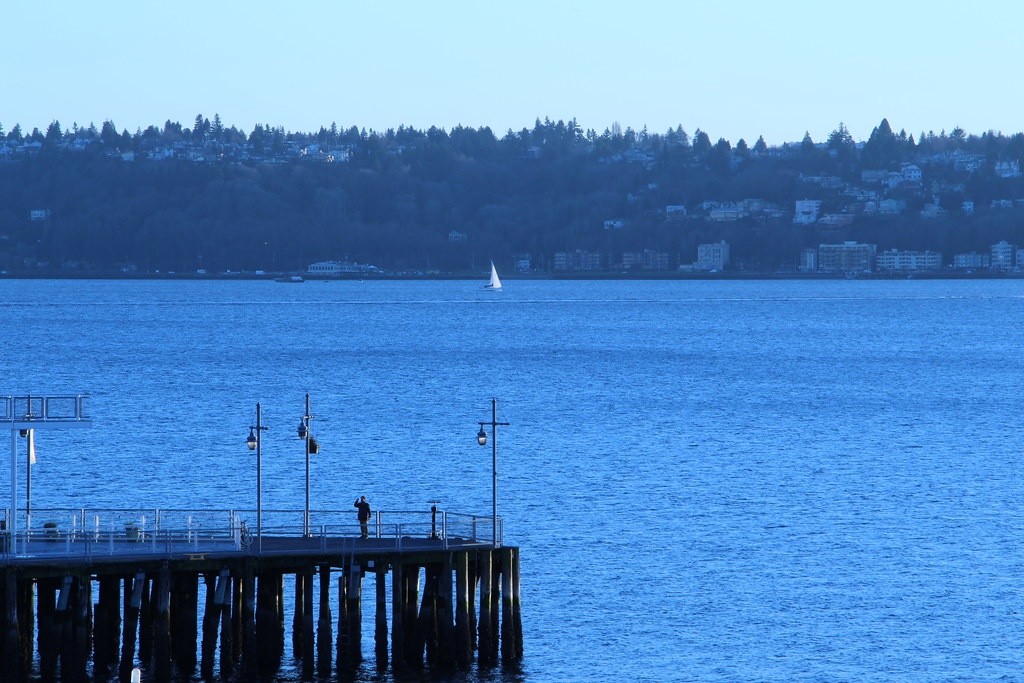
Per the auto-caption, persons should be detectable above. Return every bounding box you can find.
[354,496,371,539]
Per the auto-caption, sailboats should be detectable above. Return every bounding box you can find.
[483,258,502,290]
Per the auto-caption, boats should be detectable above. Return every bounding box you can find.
[274,277,304,282]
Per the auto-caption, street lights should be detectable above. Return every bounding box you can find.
[296,392,320,538]
[475,397,511,546]
[19,428,31,545]
[246,402,269,551]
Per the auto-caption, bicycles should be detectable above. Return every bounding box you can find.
[241,518,255,546]
